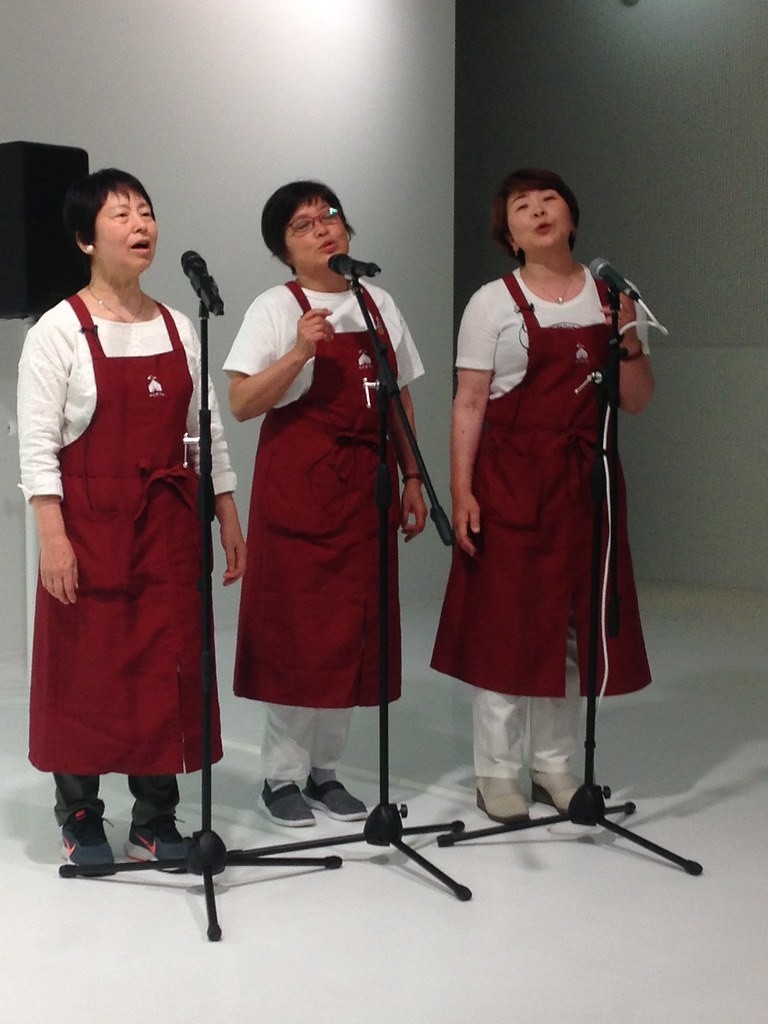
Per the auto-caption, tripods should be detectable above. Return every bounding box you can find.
[61,277,704,943]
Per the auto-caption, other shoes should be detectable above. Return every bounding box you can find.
[259,778,316,827]
[300,772,369,820]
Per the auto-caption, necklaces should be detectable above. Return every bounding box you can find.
[87,285,144,323]
[526,266,573,303]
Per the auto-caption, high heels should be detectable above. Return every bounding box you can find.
[474,777,530,824]
[527,766,578,814]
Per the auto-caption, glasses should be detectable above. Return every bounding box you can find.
[285,203,343,232]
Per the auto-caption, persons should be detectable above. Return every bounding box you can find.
[430,166,655,824]
[14,167,247,877]
[221,180,427,824]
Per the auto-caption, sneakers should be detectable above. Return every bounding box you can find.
[62,808,116,876]
[125,815,187,872]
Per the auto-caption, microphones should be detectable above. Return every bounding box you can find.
[328,254,382,278]
[589,257,641,303]
[181,249,224,316]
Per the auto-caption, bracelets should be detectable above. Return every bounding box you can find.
[402,473,423,484]
[620,349,642,362]
